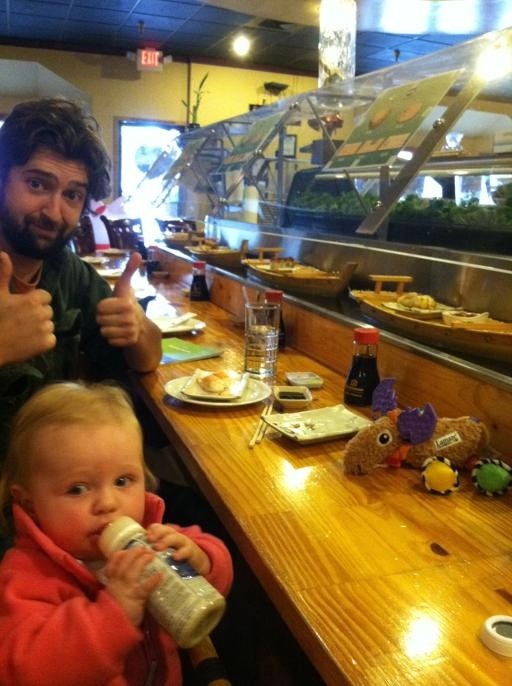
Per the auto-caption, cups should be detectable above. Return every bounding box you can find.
[242,301,281,379]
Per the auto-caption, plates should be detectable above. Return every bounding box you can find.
[81,254,128,278]
[259,403,374,446]
[257,263,297,272]
[441,309,490,327]
[381,300,464,319]
[285,370,324,388]
[151,270,169,278]
[164,373,273,407]
[179,367,250,401]
[151,316,206,334]
[273,383,314,410]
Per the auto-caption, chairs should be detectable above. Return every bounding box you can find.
[156,218,197,233]
[100,216,145,248]
[73,216,96,256]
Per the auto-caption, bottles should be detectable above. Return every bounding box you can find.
[138,236,145,251]
[147,245,159,274]
[264,288,285,350]
[344,327,382,406]
[95,514,228,649]
[190,260,210,301]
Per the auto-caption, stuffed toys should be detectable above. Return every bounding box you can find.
[344,402,512,498]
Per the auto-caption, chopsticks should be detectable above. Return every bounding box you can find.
[247,403,273,448]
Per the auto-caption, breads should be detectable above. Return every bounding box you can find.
[397,291,437,310]
[196,369,232,394]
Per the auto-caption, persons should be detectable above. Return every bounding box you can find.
[0,95,162,384]
[0,380,233,686]
